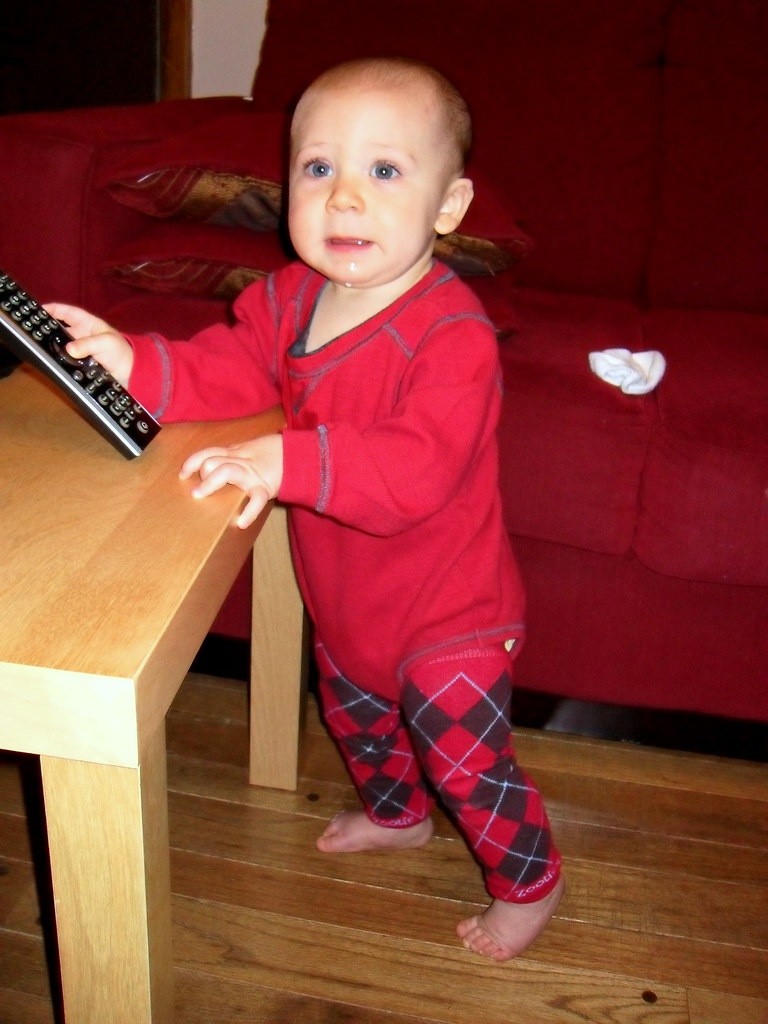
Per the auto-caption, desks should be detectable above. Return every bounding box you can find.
[0,351,311,1024]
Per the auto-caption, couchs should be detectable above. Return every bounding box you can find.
[0,0,768,718]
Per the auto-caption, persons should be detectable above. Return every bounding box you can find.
[40,58,565,962]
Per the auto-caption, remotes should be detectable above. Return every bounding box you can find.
[0,270,161,457]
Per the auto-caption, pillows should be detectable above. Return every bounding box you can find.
[100,112,539,281]
[100,223,525,340]
